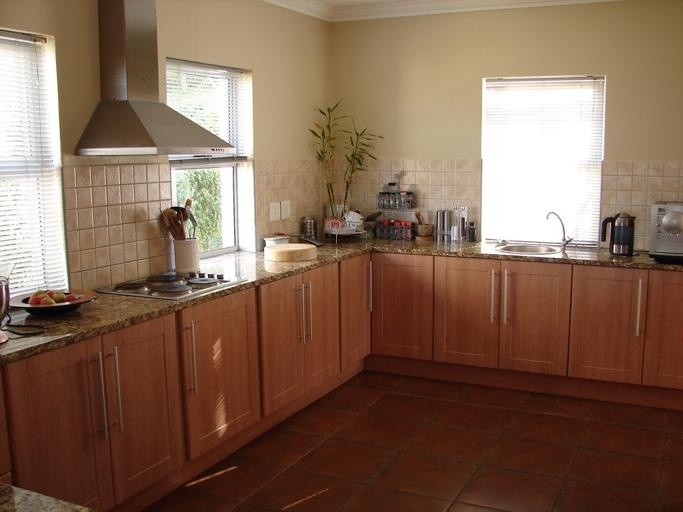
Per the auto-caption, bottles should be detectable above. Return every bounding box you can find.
[435,206,476,241]
[377,218,415,240]
[378,182,413,208]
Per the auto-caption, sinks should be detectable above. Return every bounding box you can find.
[501,245,556,255]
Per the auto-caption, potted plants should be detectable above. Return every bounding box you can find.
[305,99,385,234]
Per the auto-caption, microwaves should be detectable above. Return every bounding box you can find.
[647,200,683,263]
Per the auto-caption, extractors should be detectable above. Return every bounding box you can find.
[74,0,236,160]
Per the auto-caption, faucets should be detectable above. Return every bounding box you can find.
[546,211,573,250]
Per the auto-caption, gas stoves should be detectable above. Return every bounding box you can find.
[96,270,247,301]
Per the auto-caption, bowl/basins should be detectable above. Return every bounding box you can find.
[9,290,93,316]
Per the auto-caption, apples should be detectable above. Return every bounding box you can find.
[28,289,78,305]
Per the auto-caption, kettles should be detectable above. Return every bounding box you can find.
[601,213,635,257]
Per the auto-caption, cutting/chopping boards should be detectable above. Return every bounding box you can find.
[263,243,317,262]
[264,259,318,274]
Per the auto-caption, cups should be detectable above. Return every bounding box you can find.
[172,239,201,275]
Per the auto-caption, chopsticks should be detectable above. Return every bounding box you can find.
[160,208,175,235]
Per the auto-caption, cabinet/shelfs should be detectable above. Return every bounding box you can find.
[567,263,683,412]
[176,287,262,461]
[370,252,434,380]
[260,262,343,417]
[432,255,572,397]
[4,312,188,511]
[339,253,372,388]
